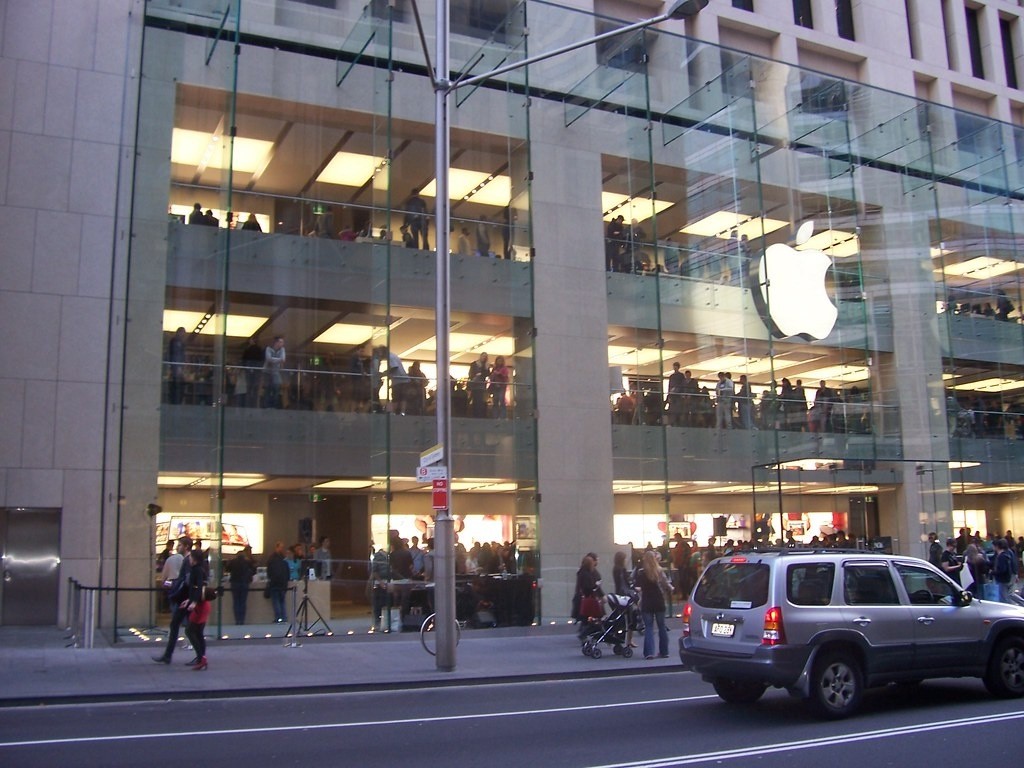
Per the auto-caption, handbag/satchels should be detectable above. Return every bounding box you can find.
[983,575,999,602]
[580,597,603,617]
[959,555,974,590]
[264,581,271,599]
[190,584,217,601]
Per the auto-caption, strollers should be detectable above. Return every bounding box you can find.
[580,586,644,659]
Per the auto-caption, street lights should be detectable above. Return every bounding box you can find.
[409,0,718,671]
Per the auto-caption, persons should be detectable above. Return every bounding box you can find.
[267,536,331,624]
[929,528,1024,606]
[169,327,509,418]
[628,531,856,601]
[945,289,1014,320]
[612,362,873,433]
[152,536,199,666]
[227,545,258,625]
[185,549,211,670]
[368,536,434,632]
[614,551,636,647]
[572,553,606,644]
[159,539,202,625]
[638,551,669,659]
[947,391,1024,440]
[608,215,752,285]
[455,541,514,575]
[190,188,491,257]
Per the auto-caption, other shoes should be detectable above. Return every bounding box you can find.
[152,655,171,664]
[272,618,288,625]
[657,653,668,658]
[646,655,654,660]
[185,657,199,666]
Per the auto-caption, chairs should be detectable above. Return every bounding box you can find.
[798,578,831,608]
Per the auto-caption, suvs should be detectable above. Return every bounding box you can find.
[677,546,1024,721]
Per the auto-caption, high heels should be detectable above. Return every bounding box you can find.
[193,655,208,670]
[626,641,638,647]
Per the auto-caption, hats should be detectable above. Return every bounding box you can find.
[391,537,402,548]
